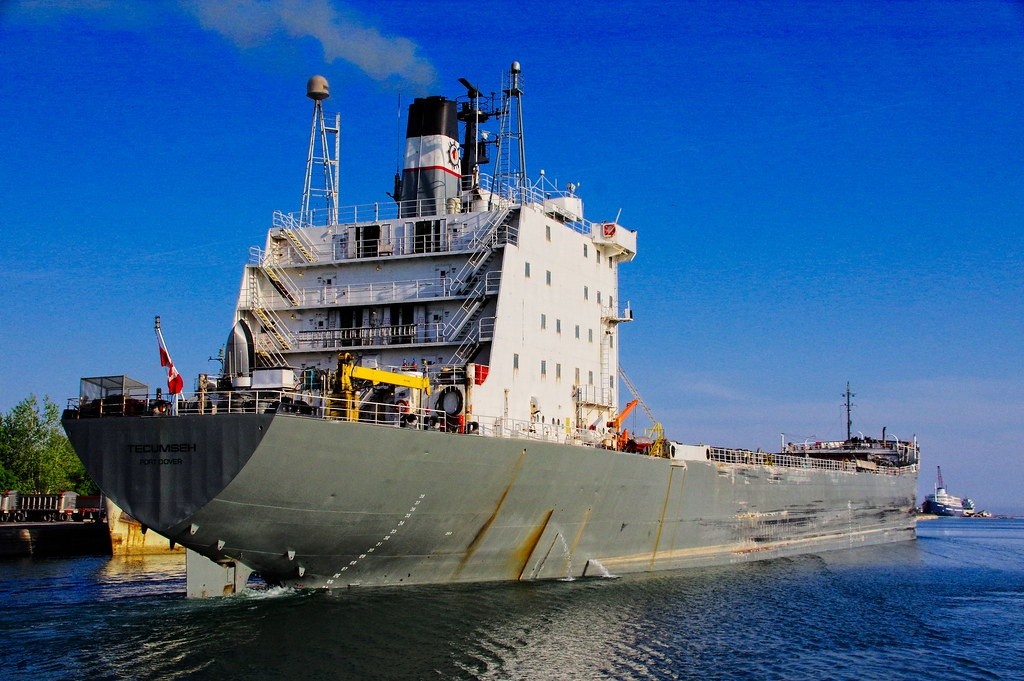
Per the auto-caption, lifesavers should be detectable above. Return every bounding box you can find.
[438,387,463,416]
[604,225,615,236]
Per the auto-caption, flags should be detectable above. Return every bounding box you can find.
[158,344,184,394]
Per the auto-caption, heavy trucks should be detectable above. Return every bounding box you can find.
[0,489,106,522]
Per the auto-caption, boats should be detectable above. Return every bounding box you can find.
[61,59,921,591]
[922,466,975,516]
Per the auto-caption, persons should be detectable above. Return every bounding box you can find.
[410,358,418,372]
[401,358,409,372]
